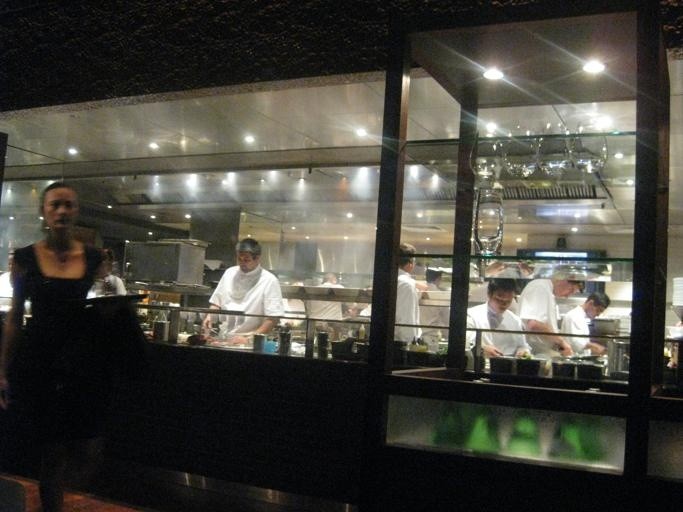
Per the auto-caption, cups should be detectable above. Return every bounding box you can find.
[467,118,610,257]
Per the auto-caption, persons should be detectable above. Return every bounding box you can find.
[0,248,34,315]
[419,268,448,290]
[465,275,531,358]
[391,242,428,349]
[0,183,108,440]
[514,263,585,358]
[308,271,353,338]
[274,277,308,329]
[200,238,284,348]
[84,270,128,300]
[560,289,610,355]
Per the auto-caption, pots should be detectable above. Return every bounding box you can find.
[607,338,631,377]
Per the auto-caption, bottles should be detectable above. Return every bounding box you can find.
[409,336,428,352]
[358,323,365,339]
